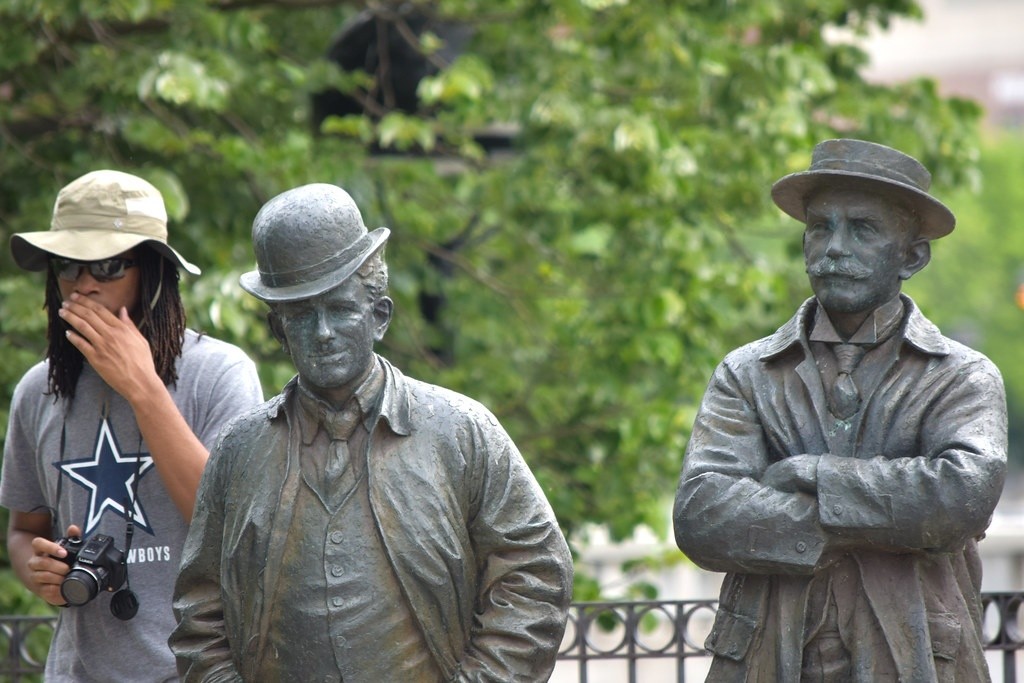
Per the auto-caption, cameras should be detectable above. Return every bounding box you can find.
[49,534,127,607]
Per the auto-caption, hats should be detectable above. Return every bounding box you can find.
[11,166,203,279]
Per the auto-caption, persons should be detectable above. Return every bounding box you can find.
[0,169,264,683]
[167,182,575,683]
[673,137,1008,683]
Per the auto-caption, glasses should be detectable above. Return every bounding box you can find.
[48,257,137,283]
